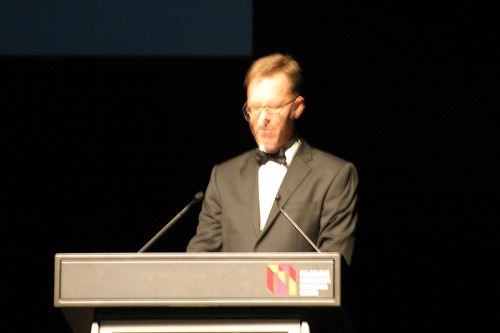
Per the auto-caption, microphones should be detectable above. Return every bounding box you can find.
[275,193,321,252]
[138,192,205,253]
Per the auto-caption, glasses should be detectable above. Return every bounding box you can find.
[242,97,299,121]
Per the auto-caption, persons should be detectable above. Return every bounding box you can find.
[184,53,363,277]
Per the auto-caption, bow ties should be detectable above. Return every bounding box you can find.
[255,148,287,165]
